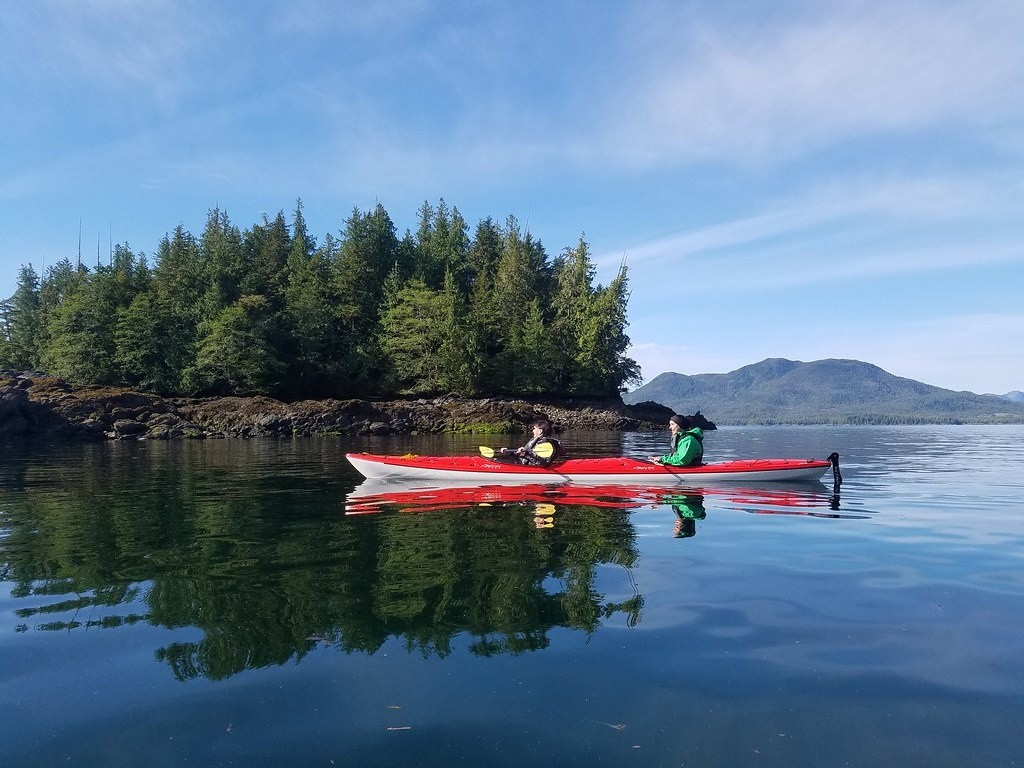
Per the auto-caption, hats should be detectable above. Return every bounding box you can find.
[670,415,689,430]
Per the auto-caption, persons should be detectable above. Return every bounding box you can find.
[501,421,556,467]
[646,415,704,466]
[652,495,707,539]
[503,502,557,529]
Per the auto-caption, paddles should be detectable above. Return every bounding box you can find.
[478,441,553,464]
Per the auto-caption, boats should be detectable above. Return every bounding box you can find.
[345,449,832,485]
